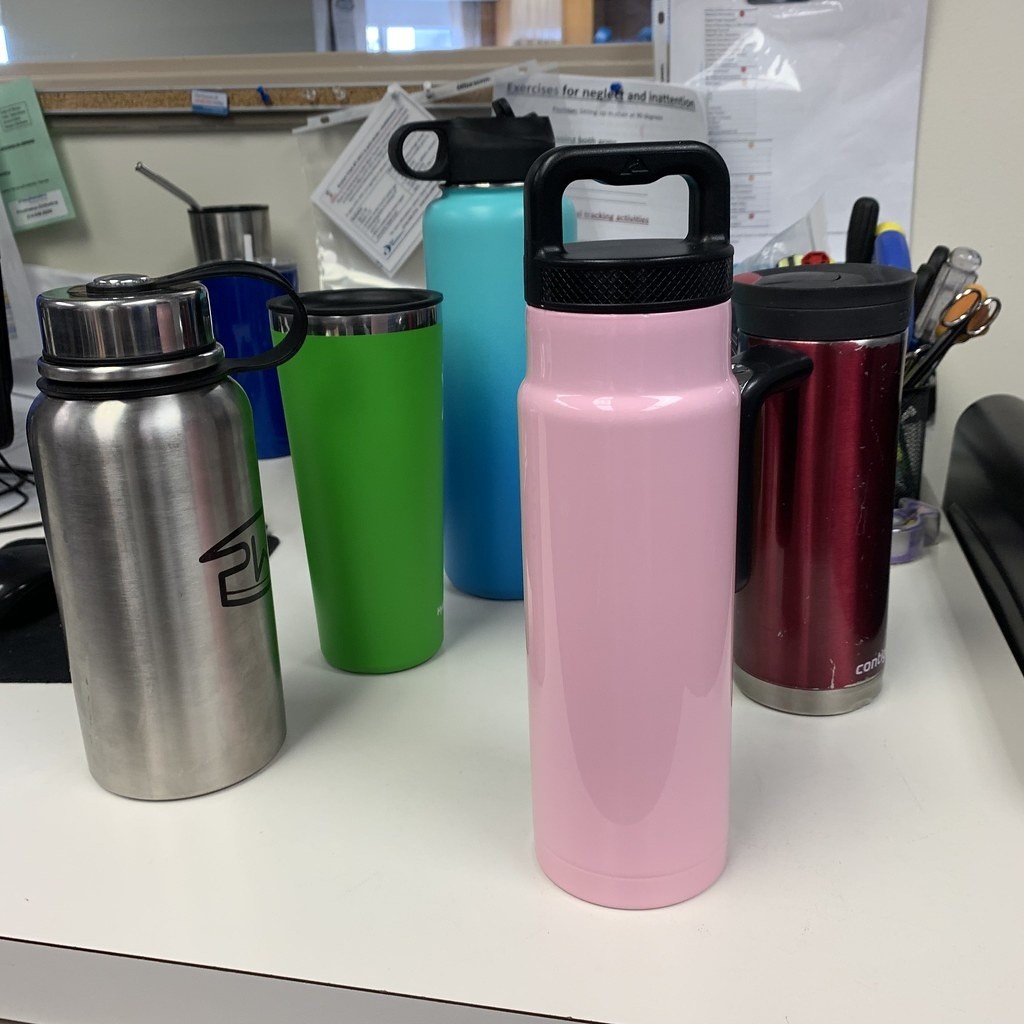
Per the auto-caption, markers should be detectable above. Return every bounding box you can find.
[798,197,911,267]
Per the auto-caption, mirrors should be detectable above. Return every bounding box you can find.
[0,0,654,115]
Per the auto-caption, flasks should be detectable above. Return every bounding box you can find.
[264,286,444,677]
[23,260,307,801]
[519,140,742,912]
[733,262,917,718]
[386,95,577,600]
[188,203,300,459]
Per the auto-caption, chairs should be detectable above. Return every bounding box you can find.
[938,397,1024,680]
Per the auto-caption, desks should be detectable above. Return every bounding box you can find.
[3,361,1017,1022]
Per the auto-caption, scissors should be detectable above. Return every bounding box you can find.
[905,245,949,330]
[903,290,1001,388]
